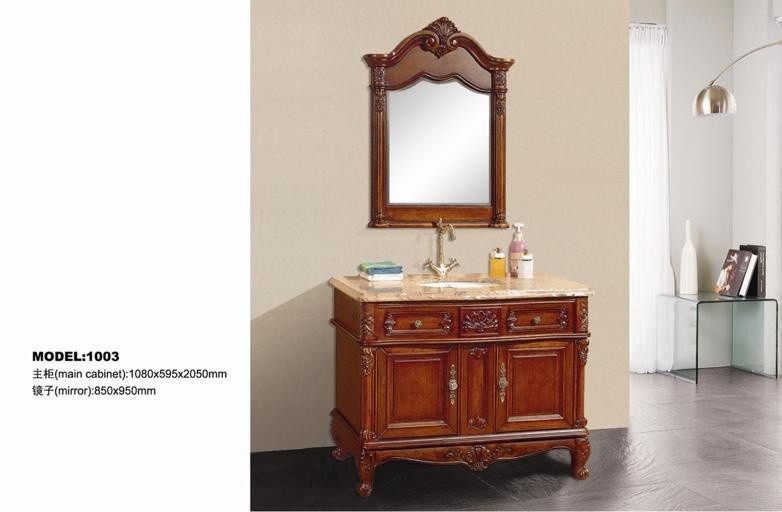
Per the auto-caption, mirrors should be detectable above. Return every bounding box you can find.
[364,14,510,231]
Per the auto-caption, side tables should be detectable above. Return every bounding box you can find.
[655,290,779,390]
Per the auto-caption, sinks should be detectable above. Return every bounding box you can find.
[412,277,506,287]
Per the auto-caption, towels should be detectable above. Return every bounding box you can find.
[359,261,404,282]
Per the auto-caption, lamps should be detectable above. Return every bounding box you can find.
[694,42,781,116]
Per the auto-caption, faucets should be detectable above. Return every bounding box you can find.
[437,224,456,267]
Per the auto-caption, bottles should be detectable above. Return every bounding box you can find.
[517,249,534,280]
[488,247,507,280]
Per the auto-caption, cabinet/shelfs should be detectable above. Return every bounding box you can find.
[331,287,591,496]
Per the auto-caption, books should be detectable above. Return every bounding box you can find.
[714,244,767,298]
